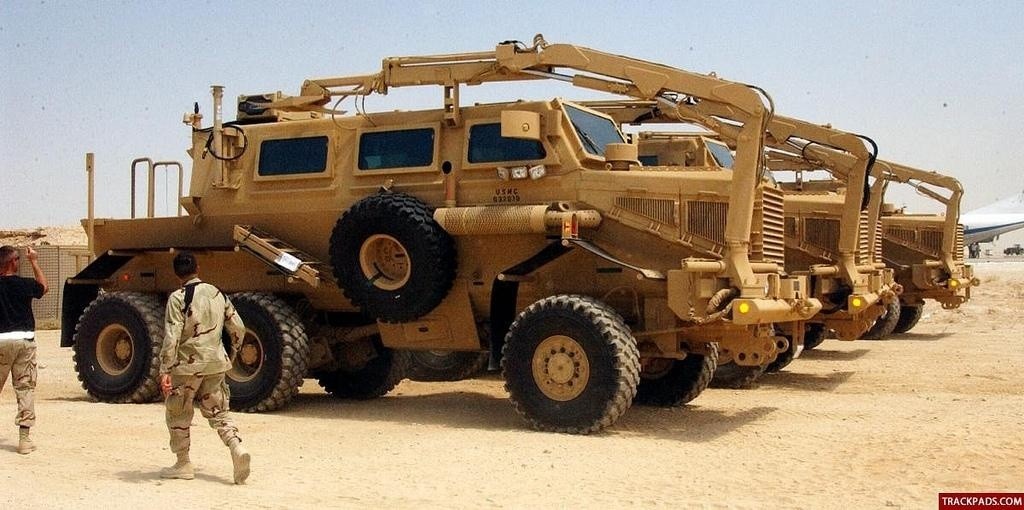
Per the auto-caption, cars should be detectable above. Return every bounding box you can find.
[1004,242,1023,257]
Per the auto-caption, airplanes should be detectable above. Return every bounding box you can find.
[956,190,1024,258]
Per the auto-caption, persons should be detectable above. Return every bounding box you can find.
[0,244,48,453]
[158,250,251,484]
[975,242,980,258]
[971,242,976,258]
[967,244,972,258]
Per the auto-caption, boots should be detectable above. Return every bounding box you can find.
[18,425,37,454]
[159,449,194,480]
[227,437,251,485]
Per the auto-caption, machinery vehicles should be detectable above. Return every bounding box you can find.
[58,47,978,433]
